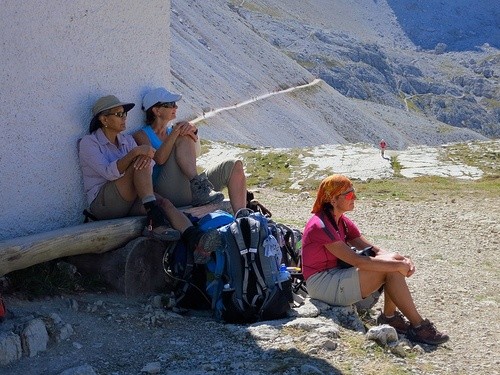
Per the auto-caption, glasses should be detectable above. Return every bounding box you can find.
[156,101,176,108]
[337,189,355,199]
[104,111,127,117]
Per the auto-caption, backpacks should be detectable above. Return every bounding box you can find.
[176,211,304,325]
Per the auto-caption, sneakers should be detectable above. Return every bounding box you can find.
[376,309,411,334]
[194,228,221,265]
[142,219,180,241]
[408,318,449,344]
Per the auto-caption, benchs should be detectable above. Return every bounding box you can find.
[0,199,232,278]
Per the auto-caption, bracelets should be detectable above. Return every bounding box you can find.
[195,127,199,135]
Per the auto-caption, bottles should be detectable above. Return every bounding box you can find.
[278,262,289,280]
[222,283,233,291]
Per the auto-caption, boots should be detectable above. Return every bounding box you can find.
[234,207,255,219]
[190,171,224,207]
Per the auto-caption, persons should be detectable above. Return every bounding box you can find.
[79,95,201,249]
[300,175,449,345]
[133,87,246,213]
[380,139,387,154]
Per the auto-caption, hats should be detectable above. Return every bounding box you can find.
[89,95,136,134]
[142,86,182,112]
